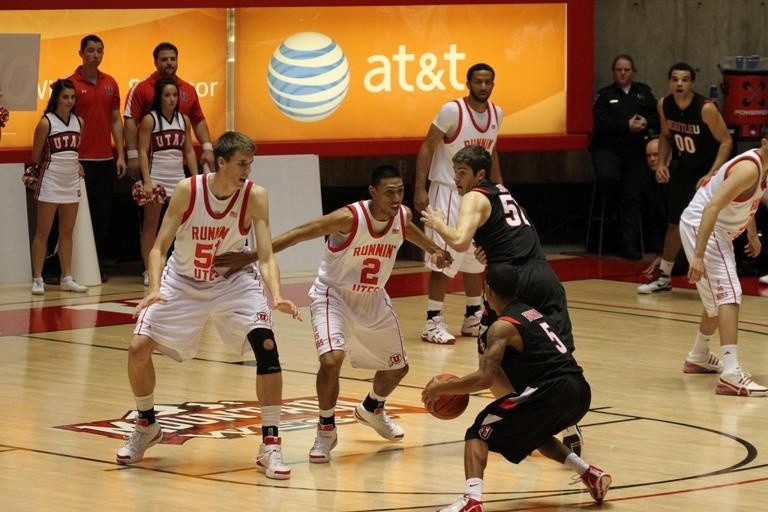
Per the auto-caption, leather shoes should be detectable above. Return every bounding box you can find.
[628,251,642,261]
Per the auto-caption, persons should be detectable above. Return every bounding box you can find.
[677,128,767,396]
[213,164,452,463]
[26,77,87,296]
[115,131,295,479]
[67,34,124,279]
[420,146,586,466]
[137,81,201,286]
[412,63,503,344]
[592,52,656,255]
[635,135,675,277]
[415,262,612,512]
[636,62,731,303]
[126,41,215,263]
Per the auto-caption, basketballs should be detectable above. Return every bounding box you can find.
[423,373,469,420]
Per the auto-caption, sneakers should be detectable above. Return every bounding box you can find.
[717,373,768,397]
[116,416,164,464]
[638,272,671,293]
[420,315,456,343]
[438,499,483,512]
[462,311,483,337]
[32,277,45,295]
[355,404,404,440]
[563,424,583,458]
[142,270,149,285]
[684,353,722,373]
[59,276,87,292]
[760,275,767,284]
[309,423,337,462]
[581,466,612,503]
[256,436,291,479]
